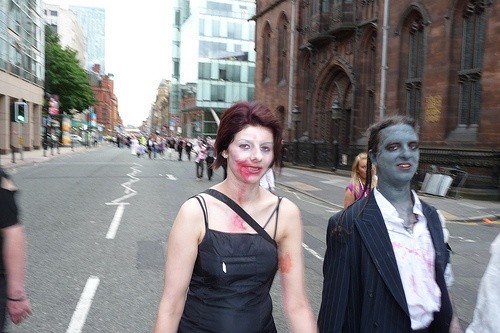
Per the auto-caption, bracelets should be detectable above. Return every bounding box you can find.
[6,297,27,302]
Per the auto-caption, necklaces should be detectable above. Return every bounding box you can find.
[377,186,414,214]
[358,177,369,198]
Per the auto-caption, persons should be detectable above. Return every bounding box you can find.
[152,101,319,333]
[280,137,286,169]
[464,234,500,333]
[0,164,35,333]
[259,167,277,196]
[316,115,463,333]
[102,128,216,181]
[341,150,377,208]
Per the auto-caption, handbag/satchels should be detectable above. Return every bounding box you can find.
[205,157,214,163]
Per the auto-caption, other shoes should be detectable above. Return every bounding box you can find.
[197,177,204,181]
